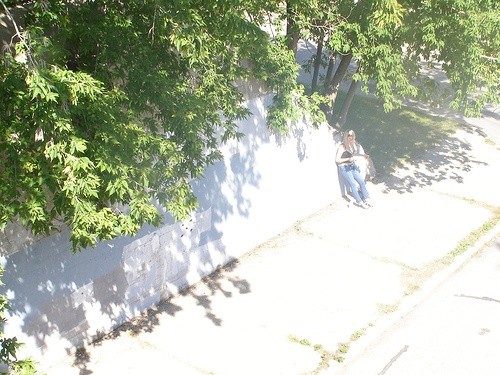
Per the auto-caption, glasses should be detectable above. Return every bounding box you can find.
[348,134,353,137]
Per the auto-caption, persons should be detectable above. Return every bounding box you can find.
[335,129,376,209]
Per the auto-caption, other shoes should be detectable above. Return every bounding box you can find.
[362,204,368,208]
[363,199,373,207]
